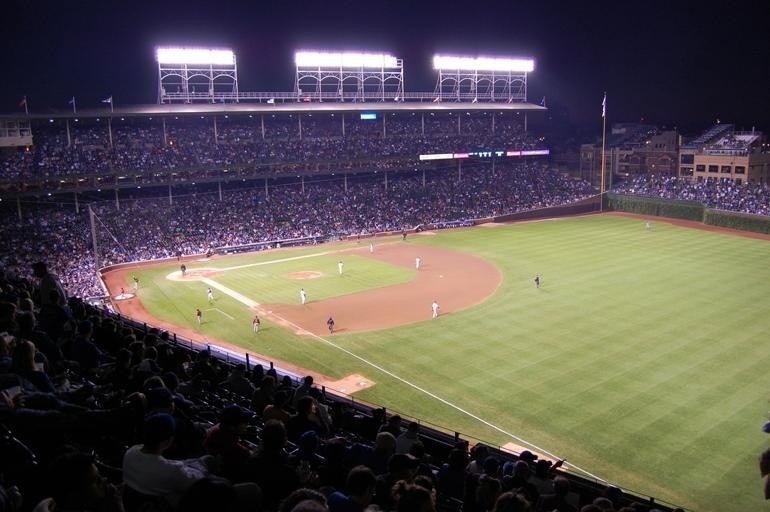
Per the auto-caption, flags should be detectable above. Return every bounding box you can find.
[601,96,607,116]
[434,95,546,104]
[21,96,26,104]
[162,95,237,104]
[103,98,111,103]
[267,95,403,104]
[69,98,73,103]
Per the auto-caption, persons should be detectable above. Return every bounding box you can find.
[687,133,770,216]
[614,123,689,199]
[0,113,604,316]
[1,282,683,512]
